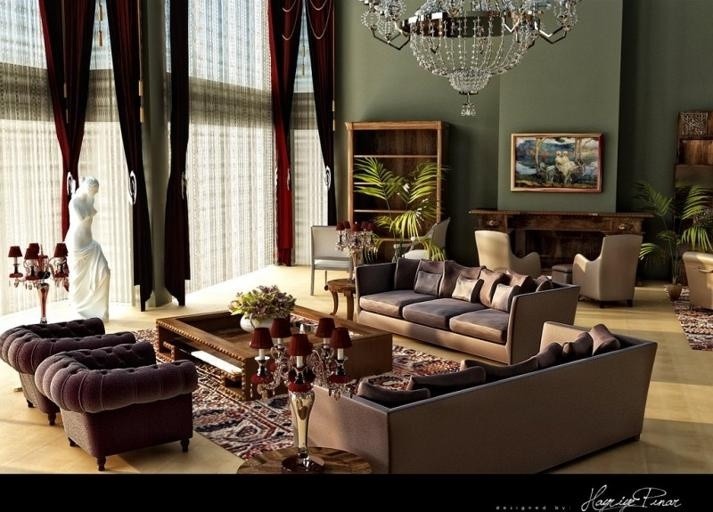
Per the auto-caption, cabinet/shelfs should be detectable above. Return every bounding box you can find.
[344,120,449,242]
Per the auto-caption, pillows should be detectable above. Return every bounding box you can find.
[393,257,553,312]
[356,323,620,409]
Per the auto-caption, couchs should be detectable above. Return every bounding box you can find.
[288,321,657,474]
[353,262,581,365]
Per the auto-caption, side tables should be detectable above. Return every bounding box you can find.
[325,279,356,320]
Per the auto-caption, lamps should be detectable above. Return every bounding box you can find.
[249,318,356,474]
[359,0,580,117]
[8,243,71,324]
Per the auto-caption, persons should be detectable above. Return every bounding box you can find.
[554,150,576,184]
[67,176,109,320]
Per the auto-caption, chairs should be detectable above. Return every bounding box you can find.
[393,218,451,259]
[0,318,198,471]
[475,230,713,311]
[311,225,354,296]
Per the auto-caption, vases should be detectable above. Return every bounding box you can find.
[239,311,292,332]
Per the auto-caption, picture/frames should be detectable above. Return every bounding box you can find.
[511,134,602,192]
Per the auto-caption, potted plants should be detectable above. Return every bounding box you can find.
[637,179,713,301]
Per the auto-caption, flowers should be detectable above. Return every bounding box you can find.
[227,285,299,330]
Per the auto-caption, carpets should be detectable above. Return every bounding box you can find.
[662,282,713,351]
[120,330,461,463]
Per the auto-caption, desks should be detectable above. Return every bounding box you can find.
[469,209,655,287]
